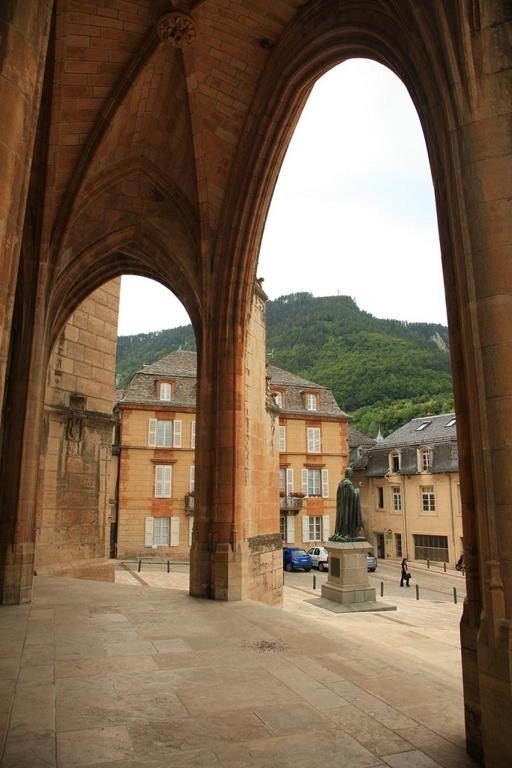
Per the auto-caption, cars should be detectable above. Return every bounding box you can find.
[306,547,330,572]
[282,547,313,571]
[366,552,378,571]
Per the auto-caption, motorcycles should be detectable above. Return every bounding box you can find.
[456,553,466,570]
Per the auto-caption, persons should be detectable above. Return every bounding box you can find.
[400,558,410,587]
[335,467,362,537]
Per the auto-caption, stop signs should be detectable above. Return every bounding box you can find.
[387,528,392,533]
